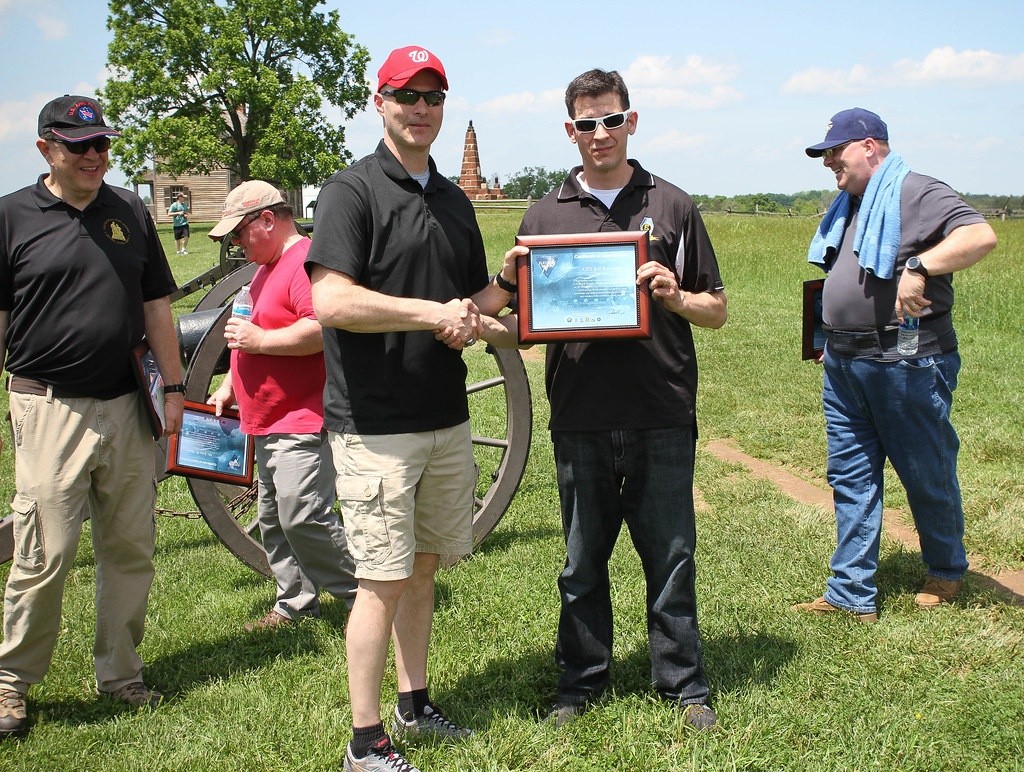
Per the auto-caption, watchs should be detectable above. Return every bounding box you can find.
[905,257,928,279]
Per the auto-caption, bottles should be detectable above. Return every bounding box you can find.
[227,286,253,343]
[897,301,918,355]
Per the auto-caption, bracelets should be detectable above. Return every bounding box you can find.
[164,382,186,395]
[497,270,517,294]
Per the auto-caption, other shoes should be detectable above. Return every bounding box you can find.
[181,249,188,255]
[177,250,181,254]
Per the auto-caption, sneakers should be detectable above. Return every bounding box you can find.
[96,682,167,710]
[1,689,30,737]
[343,736,425,772]
[541,687,607,726]
[914,577,963,608]
[243,609,292,633]
[788,594,876,627]
[682,704,717,733]
[394,703,470,739]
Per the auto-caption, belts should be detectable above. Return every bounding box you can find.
[7,376,139,401]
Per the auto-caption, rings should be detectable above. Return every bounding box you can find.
[663,277,668,287]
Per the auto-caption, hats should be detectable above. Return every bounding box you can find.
[806,108,890,158]
[376,45,450,93]
[177,193,187,200]
[38,93,123,141]
[208,179,284,242]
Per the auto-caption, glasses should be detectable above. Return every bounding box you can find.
[381,89,446,109]
[571,110,630,133]
[820,140,852,159]
[227,214,262,237]
[45,138,111,155]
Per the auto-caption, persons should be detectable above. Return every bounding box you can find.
[0,94,183,737]
[433,66,728,729]
[168,192,190,255]
[208,179,359,632]
[786,106,997,623]
[303,44,529,772]
